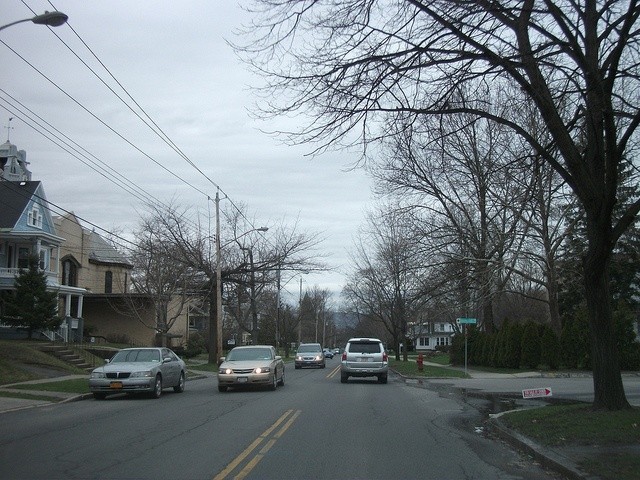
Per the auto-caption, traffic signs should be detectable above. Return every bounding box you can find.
[521,386,552,399]
[456,317,478,325]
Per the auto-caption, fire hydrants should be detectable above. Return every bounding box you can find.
[416,353,424,370]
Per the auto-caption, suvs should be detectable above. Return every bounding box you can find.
[340,336,388,384]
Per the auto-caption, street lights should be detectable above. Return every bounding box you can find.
[0,8,71,37]
[217,226,269,362]
[274,269,310,352]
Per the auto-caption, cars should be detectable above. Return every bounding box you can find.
[293,343,325,369]
[217,345,285,392]
[323,344,340,359]
[88,346,185,400]
